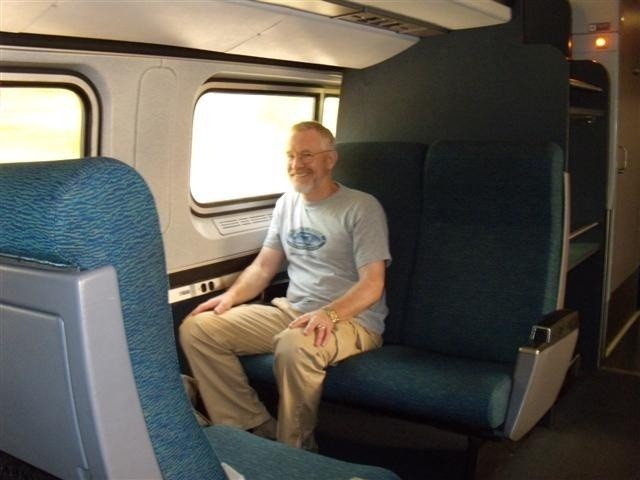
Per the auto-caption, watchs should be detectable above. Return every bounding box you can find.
[320,306,339,324]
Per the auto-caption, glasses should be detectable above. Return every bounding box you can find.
[284,150,331,162]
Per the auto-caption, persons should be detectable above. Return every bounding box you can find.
[178,122,393,454]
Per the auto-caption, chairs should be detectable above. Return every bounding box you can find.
[348,136,582,479]
[237,140,429,411]
[2,155,400,479]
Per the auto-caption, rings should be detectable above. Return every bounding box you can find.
[317,326,327,330]
